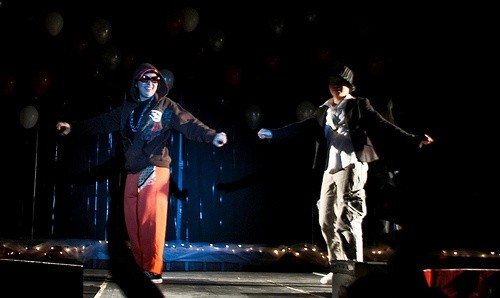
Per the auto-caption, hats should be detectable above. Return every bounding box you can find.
[135,67,159,79]
[322,65,356,92]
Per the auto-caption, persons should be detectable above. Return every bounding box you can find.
[55,62,228,285]
[257,65,435,285]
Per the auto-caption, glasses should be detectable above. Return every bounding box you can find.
[137,75,160,83]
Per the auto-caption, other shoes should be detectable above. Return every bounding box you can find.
[319,271,334,285]
[145,272,163,284]
